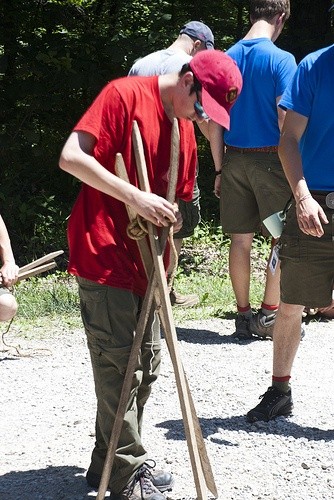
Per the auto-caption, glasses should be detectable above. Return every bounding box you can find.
[194,78,214,123]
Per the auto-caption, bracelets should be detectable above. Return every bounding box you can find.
[215,168,221,176]
[293,191,311,207]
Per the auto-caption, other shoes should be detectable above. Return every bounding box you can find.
[169,289,199,309]
[86,459,175,491]
[110,467,168,500]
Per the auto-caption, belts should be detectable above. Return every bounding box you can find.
[223,144,280,154]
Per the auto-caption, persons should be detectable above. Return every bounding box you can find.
[0,214,26,323]
[126,21,214,307]
[246,40,334,424]
[207,0,307,341]
[58,49,242,499]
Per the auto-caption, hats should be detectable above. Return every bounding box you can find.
[189,49,243,131]
[179,20,215,50]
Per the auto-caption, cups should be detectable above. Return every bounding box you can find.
[261,210,288,239]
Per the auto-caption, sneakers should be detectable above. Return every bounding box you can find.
[246,387,293,423]
[234,315,254,340]
[247,310,306,341]
